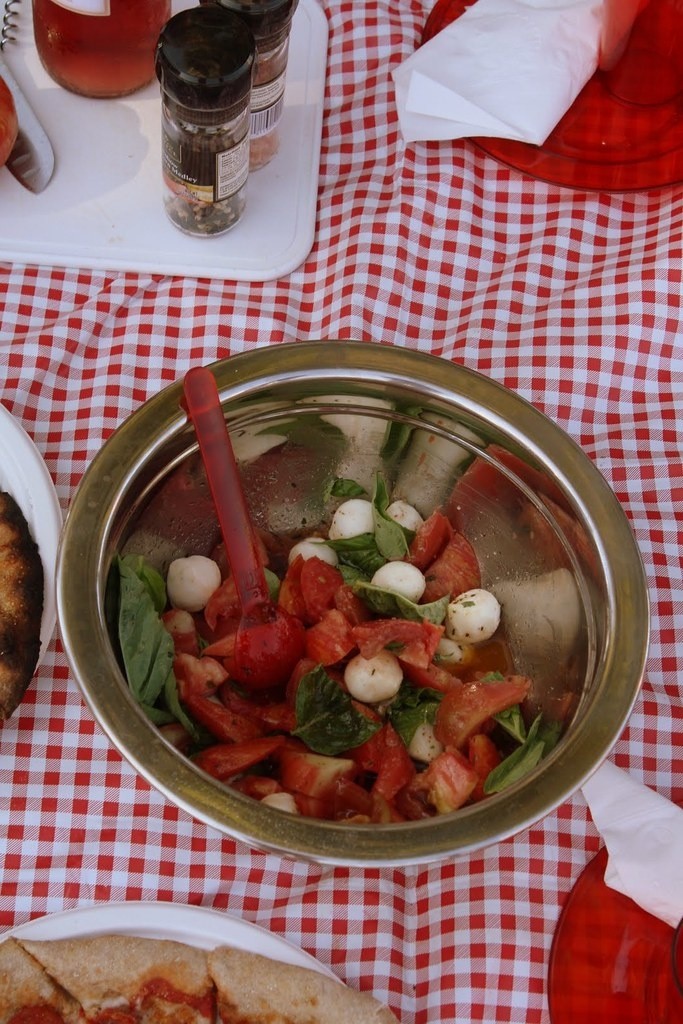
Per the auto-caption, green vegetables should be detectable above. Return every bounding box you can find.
[112,469,548,797]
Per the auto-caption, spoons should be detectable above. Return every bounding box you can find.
[185,366,304,693]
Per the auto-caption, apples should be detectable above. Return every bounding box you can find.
[0,76,19,169]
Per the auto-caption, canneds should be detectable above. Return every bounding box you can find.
[31,0,172,98]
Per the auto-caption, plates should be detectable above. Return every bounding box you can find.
[421,0,683,192]
[0,405,60,674]
[0,901,350,990]
[548,843,683,1024]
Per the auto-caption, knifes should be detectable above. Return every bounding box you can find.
[0,55,54,194]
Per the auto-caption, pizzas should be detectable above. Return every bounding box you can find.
[0,935,400,1024]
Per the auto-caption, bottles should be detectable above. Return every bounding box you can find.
[199,0,299,171]
[32,0,172,98]
[154,6,257,235]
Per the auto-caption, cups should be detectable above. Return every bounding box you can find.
[602,0,683,107]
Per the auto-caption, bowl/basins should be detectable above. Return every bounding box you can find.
[54,340,651,865]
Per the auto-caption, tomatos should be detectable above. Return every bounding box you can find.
[164,513,532,825]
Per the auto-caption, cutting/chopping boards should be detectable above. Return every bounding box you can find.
[1,0,330,281]
